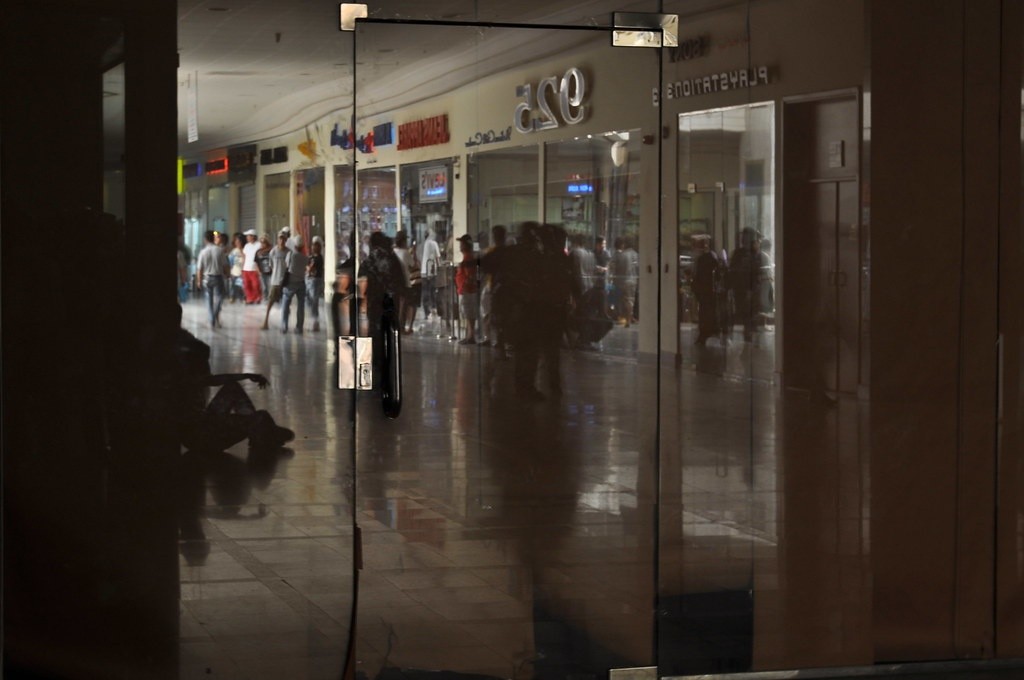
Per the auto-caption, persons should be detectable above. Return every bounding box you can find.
[178,224,325,336]
[692,227,775,345]
[567,227,639,328]
[340,220,577,416]
[177,303,296,452]
[177,442,271,567]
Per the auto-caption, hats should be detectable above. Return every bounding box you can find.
[312,236,323,247]
[243,229,257,235]
[282,226,290,236]
[392,230,409,242]
[456,234,472,243]
[278,231,287,240]
[293,235,304,248]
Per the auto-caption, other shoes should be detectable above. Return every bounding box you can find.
[260,325,269,330]
[493,342,506,348]
[479,340,491,347]
[399,327,413,334]
[459,337,475,345]
